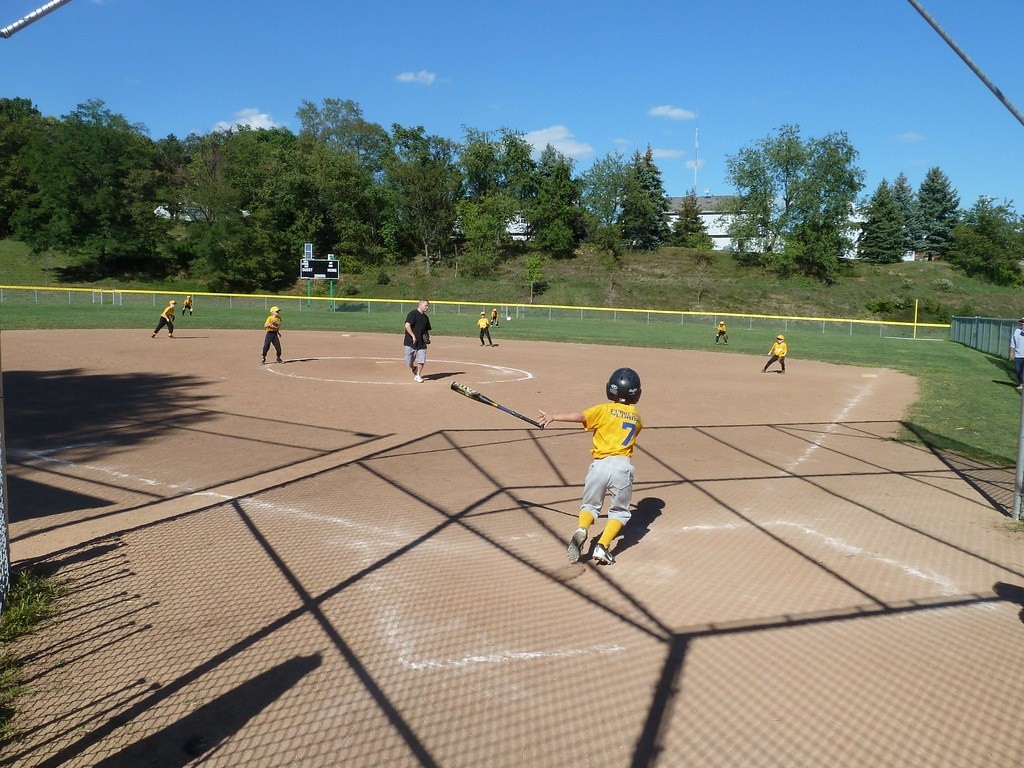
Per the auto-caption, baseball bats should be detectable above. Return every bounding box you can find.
[450,381,545,430]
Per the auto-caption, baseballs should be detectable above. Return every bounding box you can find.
[507,317,512,321]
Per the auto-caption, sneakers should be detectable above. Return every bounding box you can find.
[412,366,417,375]
[414,375,424,383]
[593,543,615,565]
[567,528,588,563]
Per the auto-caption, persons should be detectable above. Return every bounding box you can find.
[151,300,177,338]
[715,321,728,345]
[490,309,499,327]
[537,368,643,565]
[182,296,193,316]
[1009,318,1024,390]
[403,299,432,383]
[761,335,787,374]
[477,312,494,347]
[261,306,285,364]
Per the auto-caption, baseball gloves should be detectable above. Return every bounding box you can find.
[270,322,281,328]
[779,356,785,364]
[723,334,728,340]
[171,315,175,322]
[422,333,430,344]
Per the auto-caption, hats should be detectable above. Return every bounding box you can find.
[1018,316,1024,323]
[720,321,724,324]
[270,306,281,312]
[480,312,485,315]
[187,296,190,298]
[169,300,176,304]
[493,309,497,312]
[776,335,784,340]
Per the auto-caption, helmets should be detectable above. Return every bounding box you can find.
[606,367,641,404]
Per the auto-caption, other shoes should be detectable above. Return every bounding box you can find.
[261,358,265,364]
[491,325,492,327]
[780,371,785,375]
[481,344,484,346]
[169,335,175,339]
[151,334,155,338]
[496,326,498,327]
[276,359,284,363]
[490,343,493,346]
[762,370,765,373]
[1017,385,1022,389]
